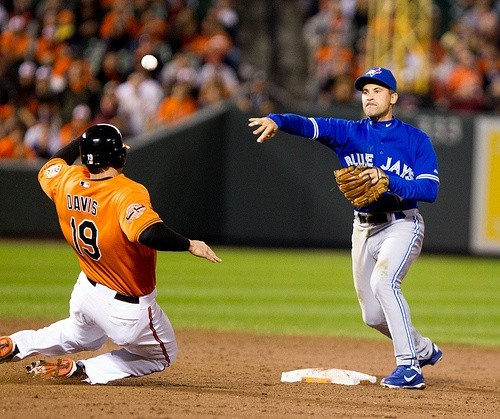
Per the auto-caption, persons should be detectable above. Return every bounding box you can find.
[0,0,500,164]
[247,66,444,390]
[0,124,223,385]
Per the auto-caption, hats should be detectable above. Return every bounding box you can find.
[355,67,397,92]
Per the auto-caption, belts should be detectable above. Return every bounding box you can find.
[86,276,139,304]
[358,211,406,224]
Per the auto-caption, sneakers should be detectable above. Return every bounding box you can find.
[380,365,426,389]
[419,343,443,368]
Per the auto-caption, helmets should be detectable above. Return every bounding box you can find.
[79,123,130,164]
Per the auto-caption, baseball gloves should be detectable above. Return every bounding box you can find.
[332,164,391,209]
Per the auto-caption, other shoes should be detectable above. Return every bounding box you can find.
[0,336,20,364]
[26,357,84,379]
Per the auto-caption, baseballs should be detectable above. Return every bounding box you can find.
[140,54,158,71]
